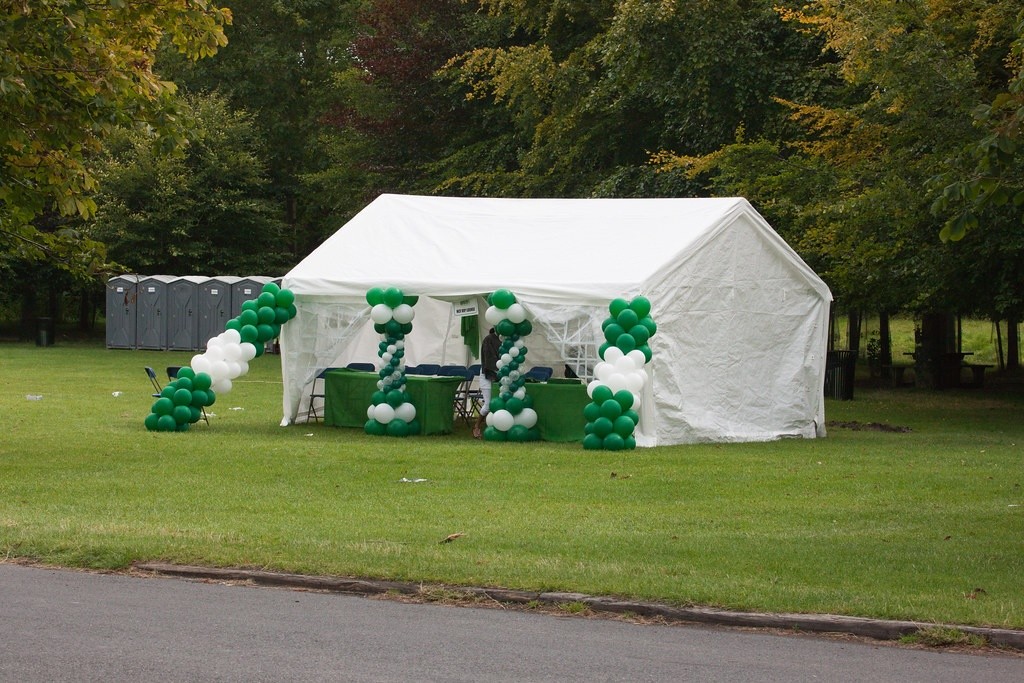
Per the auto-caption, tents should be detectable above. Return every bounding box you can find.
[280,193,834,448]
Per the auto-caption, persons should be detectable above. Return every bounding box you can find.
[472,328,502,440]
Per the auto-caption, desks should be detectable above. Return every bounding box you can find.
[325,368,466,435]
[522,377,595,384]
[490,383,594,442]
[903,353,973,388]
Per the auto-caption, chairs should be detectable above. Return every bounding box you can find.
[145,366,163,398]
[167,366,209,426]
[521,372,550,382]
[347,363,375,372]
[406,367,423,374]
[530,366,554,377]
[457,365,484,427]
[447,370,474,426]
[306,367,346,422]
[438,366,467,410]
[416,364,440,375]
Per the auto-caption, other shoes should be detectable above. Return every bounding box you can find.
[472,427,484,439]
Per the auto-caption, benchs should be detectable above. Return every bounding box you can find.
[883,364,914,389]
[963,364,995,390]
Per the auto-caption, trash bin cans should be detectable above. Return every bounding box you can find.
[824,350,857,401]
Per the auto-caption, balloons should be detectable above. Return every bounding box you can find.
[144,283,297,434]
[365,287,420,436]
[582,296,657,450]
[484,289,542,443]
